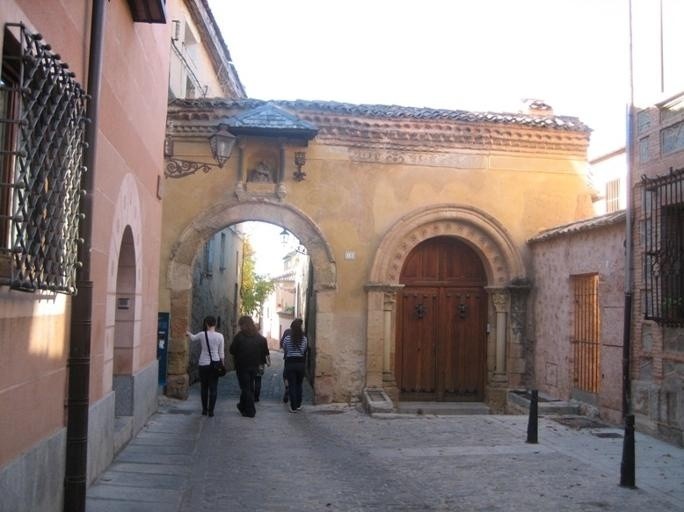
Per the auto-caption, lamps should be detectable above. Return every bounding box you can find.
[291,150,308,183]
[163,122,237,179]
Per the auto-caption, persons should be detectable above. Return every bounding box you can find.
[253,323,271,403]
[184,316,225,417]
[280,318,307,412]
[229,315,265,417]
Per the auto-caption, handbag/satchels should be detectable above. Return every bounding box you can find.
[210,359,227,377]
